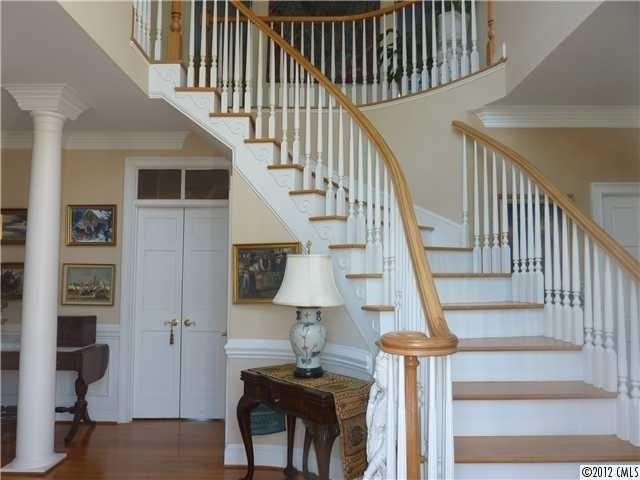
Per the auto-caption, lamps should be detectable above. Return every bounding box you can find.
[274,252,346,378]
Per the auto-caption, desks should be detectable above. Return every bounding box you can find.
[236,363,370,480]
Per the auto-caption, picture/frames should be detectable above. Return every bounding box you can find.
[0,208,29,245]
[62,264,116,305]
[0,263,25,299]
[64,204,117,245]
[229,241,300,305]
